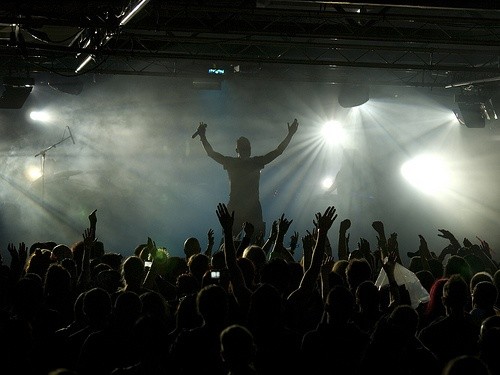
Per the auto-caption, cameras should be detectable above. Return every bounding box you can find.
[210,270,222,278]
[144,260,152,268]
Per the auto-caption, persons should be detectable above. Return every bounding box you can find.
[0,202,500,375]
[198,119,299,248]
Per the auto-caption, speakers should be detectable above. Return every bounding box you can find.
[458,102,486,128]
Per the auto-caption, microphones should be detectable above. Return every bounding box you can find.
[67,126,75,144]
[192,124,207,138]
